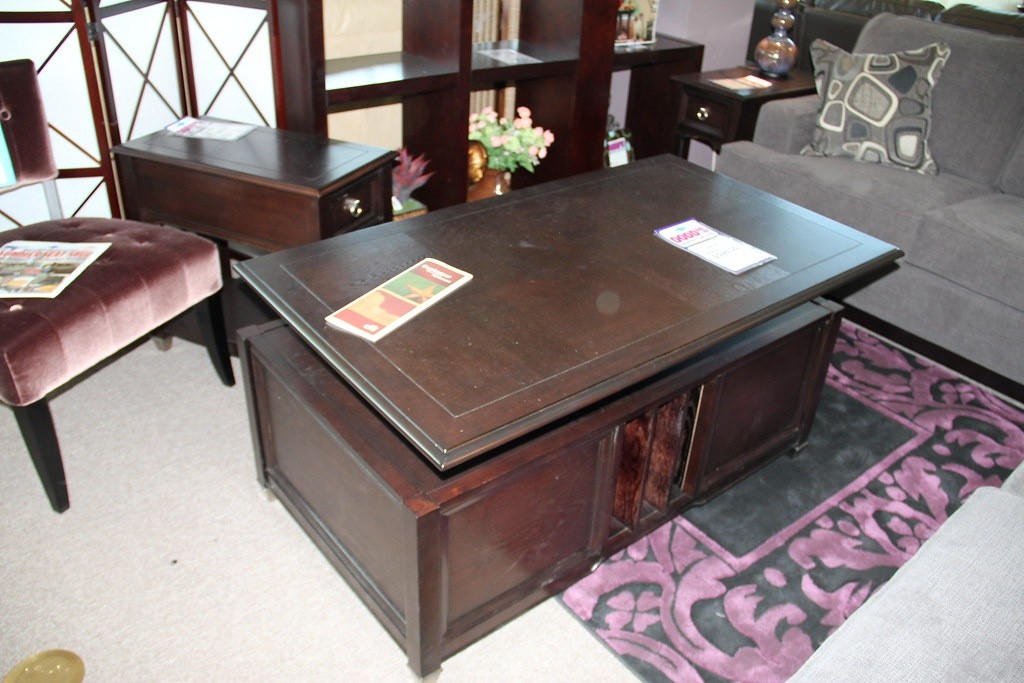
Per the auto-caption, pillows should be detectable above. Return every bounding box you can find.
[799,37,951,177]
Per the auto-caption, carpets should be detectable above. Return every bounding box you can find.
[558,318,1024,683]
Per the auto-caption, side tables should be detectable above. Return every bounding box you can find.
[670,64,817,162]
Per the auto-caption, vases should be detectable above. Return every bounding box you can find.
[466,169,513,204]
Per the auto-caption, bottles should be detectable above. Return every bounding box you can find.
[607,115,630,168]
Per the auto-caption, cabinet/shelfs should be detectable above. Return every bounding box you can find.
[272,0,705,208]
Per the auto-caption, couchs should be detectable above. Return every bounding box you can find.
[716,11,1024,403]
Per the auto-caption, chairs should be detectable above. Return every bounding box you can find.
[0,58,236,515]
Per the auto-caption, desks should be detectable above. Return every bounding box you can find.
[106,110,402,360]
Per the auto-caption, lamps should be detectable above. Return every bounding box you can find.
[754,0,801,78]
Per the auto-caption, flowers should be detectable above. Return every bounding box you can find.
[470,106,556,171]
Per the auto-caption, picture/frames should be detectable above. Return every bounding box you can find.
[603,127,635,170]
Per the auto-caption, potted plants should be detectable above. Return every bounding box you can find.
[390,148,436,221]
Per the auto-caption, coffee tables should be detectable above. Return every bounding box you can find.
[235,151,908,683]
